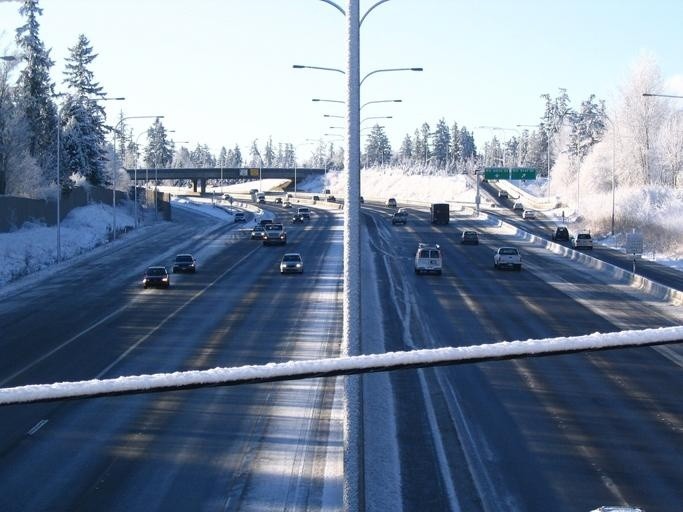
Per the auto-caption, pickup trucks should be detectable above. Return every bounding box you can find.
[494,247,522,270]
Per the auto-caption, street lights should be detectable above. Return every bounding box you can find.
[112,115,175,238]
[577,112,617,235]
[642,93,682,99]
[293,63,422,138]
[56,97,126,260]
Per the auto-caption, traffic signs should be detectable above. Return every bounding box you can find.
[512,168,536,180]
[485,167,509,180]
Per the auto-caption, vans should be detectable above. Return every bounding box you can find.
[414,246,443,275]
[297,207,309,219]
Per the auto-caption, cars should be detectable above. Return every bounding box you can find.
[571,234,593,249]
[552,227,568,241]
[385,198,396,206]
[292,214,303,224]
[513,202,522,210]
[313,195,335,202]
[172,254,196,274]
[275,198,292,208]
[143,267,169,290]
[524,210,534,219]
[498,190,508,198]
[279,253,304,274]
[392,208,408,224]
[234,213,245,221]
[252,220,272,239]
[462,230,479,245]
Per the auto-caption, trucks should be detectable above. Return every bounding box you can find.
[430,203,450,225]
[255,192,265,204]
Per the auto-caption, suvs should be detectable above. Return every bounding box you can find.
[263,224,286,246]
